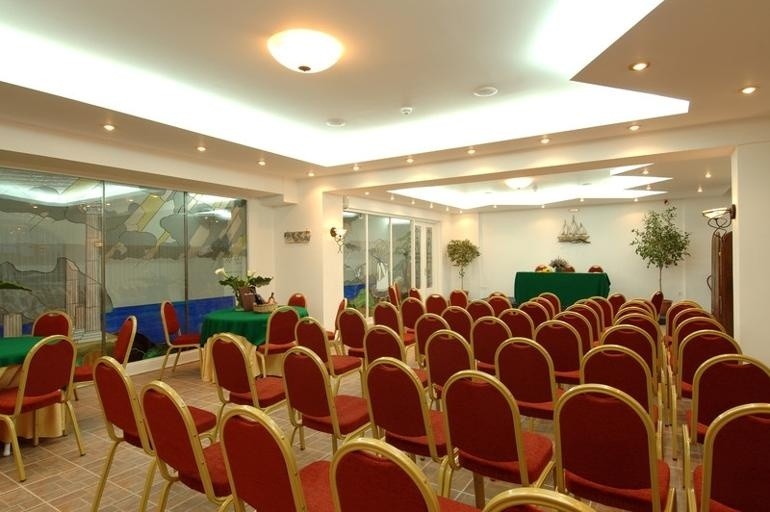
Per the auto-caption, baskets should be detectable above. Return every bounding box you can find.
[254,304,277,313]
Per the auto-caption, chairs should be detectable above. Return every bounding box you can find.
[92,356,217,512]
[563,265,575,272]
[256,308,300,378]
[207,333,306,452]
[287,294,306,308]
[324,284,769,512]
[32,310,78,400]
[1,335,87,483]
[138,380,233,511]
[294,317,363,396]
[159,300,204,379]
[588,264,603,272]
[280,345,371,454]
[73,316,138,400]
[535,265,549,272]
[218,406,337,511]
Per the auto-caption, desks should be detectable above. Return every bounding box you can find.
[1,336,42,365]
[511,272,612,305]
[205,306,307,323]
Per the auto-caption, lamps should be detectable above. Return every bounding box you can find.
[503,176,534,190]
[267,26,347,77]
[330,227,347,253]
[702,204,735,239]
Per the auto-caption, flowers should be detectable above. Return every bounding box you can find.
[214,267,274,309]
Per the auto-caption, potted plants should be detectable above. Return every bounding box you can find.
[447,239,483,296]
[629,206,692,316]
